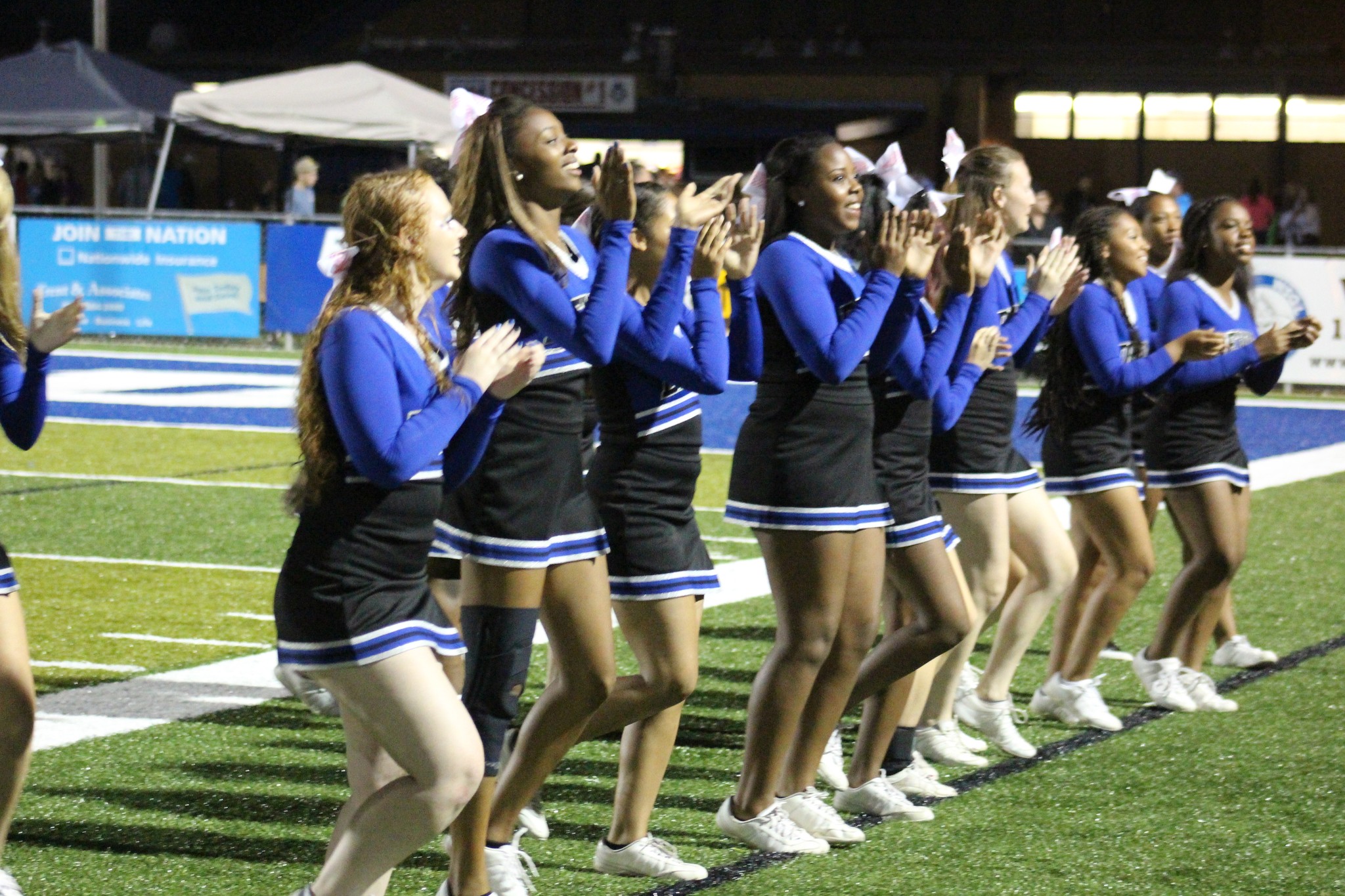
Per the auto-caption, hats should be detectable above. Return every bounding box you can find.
[293,155,319,173]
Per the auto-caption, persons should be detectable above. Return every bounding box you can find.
[1017,190,1058,238]
[0,173,83,896]
[1237,178,1276,244]
[1284,185,1320,242]
[502,179,769,885]
[1017,207,1224,732]
[441,97,638,896]
[812,146,1091,825]
[1130,192,1322,711]
[287,156,319,215]
[1098,190,1278,666]
[715,133,945,855]
[274,167,545,896]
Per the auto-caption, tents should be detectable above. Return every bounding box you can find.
[142,64,460,206]
[2,41,286,155]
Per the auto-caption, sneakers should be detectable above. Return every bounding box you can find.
[443,834,528,896]
[934,716,989,753]
[498,727,551,839]
[816,728,850,792]
[714,794,830,855]
[911,749,940,780]
[773,791,866,844]
[880,763,958,797]
[832,775,935,821]
[952,688,1038,758]
[913,727,989,767]
[1177,669,1239,712]
[1027,686,1081,724]
[274,662,340,718]
[592,836,709,880]
[1129,645,1197,712]
[1214,634,1277,668]
[1041,671,1123,731]
